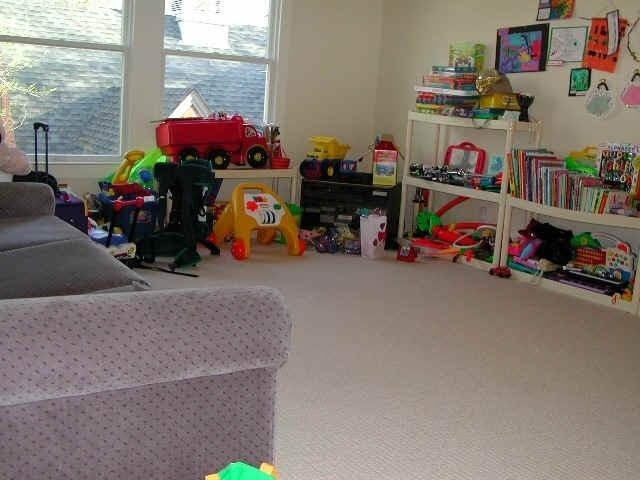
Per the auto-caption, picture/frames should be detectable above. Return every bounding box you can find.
[495,22,549,73]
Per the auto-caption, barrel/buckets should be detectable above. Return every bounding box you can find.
[360,214,386,259]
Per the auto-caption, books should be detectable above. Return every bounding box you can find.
[506,144,633,217]
[415,65,480,117]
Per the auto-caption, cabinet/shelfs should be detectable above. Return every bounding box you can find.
[300,172,403,250]
[397,110,541,273]
[499,191,640,316]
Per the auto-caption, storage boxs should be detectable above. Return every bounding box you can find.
[98,191,158,238]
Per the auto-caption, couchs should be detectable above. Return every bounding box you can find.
[1,181,293,480]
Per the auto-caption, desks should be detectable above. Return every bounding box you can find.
[210,168,301,241]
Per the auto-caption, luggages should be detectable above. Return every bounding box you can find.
[12,121,57,192]
[55,188,89,235]
